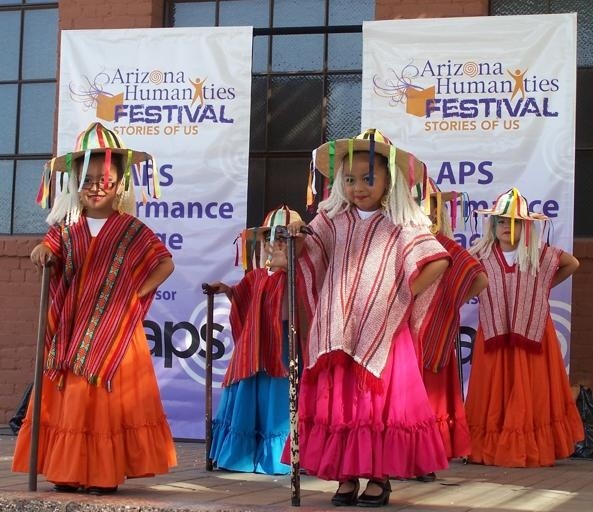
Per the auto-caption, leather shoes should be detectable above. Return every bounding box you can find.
[53,485,118,496]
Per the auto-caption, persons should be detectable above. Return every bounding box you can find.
[463,189,586,467]
[410,177,488,482]
[209,205,303,475]
[11,122,177,493]
[287,130,453,507]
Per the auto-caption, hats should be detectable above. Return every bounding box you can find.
[43,122,152,172]
[473,187,550,221]
[239,204,306,241]
[312,128,463,200]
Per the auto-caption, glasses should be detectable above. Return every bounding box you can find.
[78,181,117,189]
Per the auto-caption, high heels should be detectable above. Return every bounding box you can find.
[357,478,392,506]
[331,478,360,506]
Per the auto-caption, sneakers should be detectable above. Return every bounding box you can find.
[417,471,436,482]
